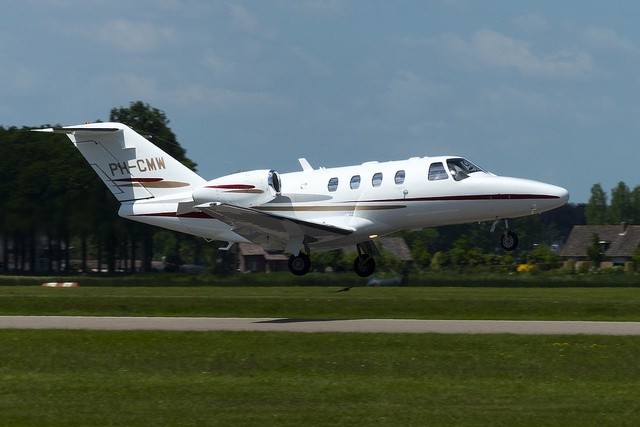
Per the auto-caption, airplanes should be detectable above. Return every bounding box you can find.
[31,122,569,278]
